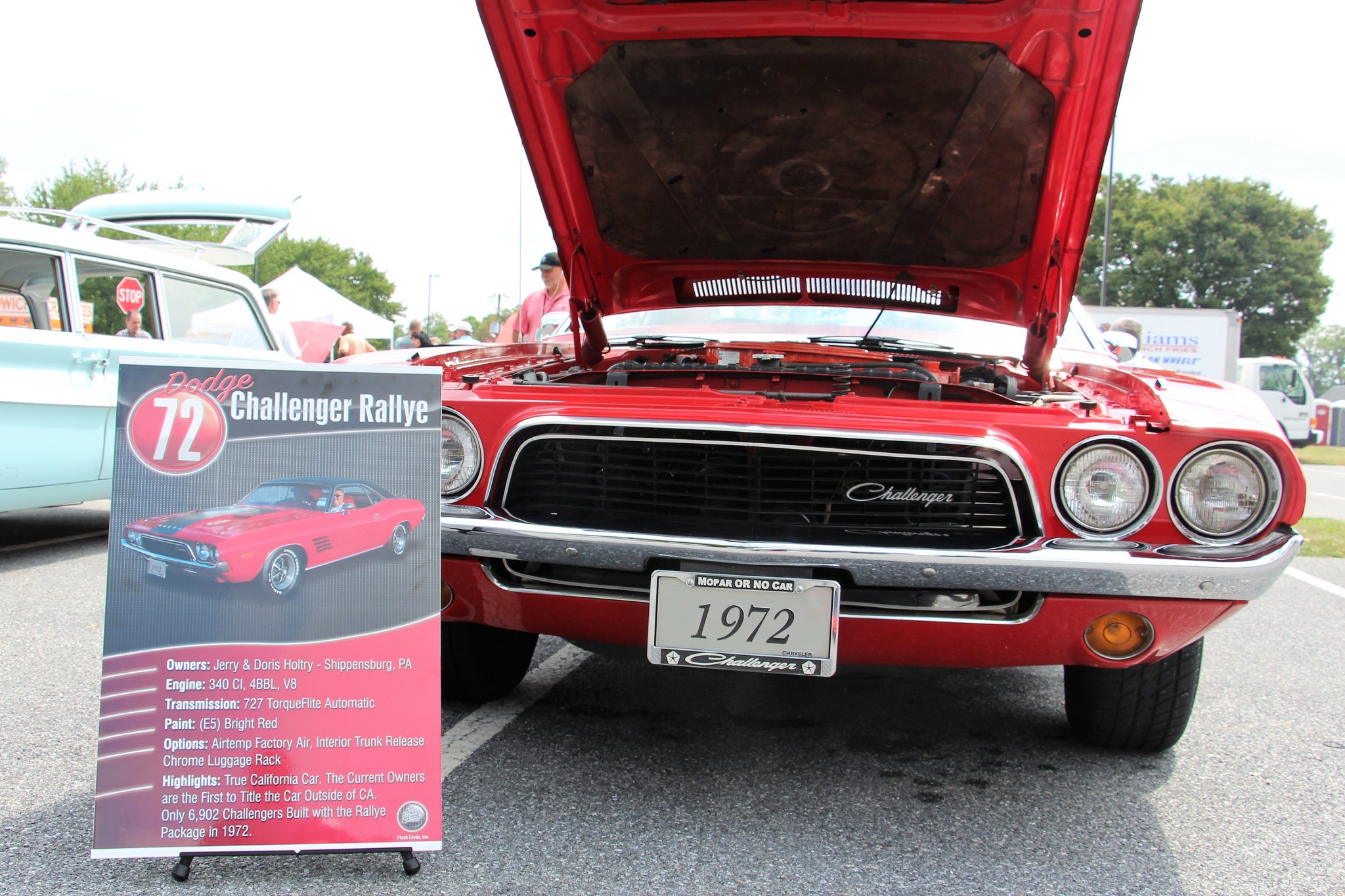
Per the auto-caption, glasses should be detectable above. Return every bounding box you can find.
[274,300,281,305]
[334,494,343,497]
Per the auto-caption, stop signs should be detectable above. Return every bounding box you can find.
[116,276,143,315]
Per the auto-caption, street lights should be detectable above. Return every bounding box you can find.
[427,274,441,337]
[496,294,510,332]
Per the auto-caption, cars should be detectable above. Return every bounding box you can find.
[123,480,426,597]
[327,0,1308,755]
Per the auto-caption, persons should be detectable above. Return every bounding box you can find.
[116,310,152,339]
[512,252,585,343]
[394,319,497,349]
[230,288,303,361]
[330,489,345,512]
[338,322,377,358]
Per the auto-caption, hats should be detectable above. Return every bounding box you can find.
[449,320,473,333]
[531,251,562,271]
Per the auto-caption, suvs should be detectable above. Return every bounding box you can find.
[0,191,296,523]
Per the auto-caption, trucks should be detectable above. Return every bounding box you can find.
[1078,305,1325,450]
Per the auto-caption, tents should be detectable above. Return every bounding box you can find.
[189,265,395,363]
